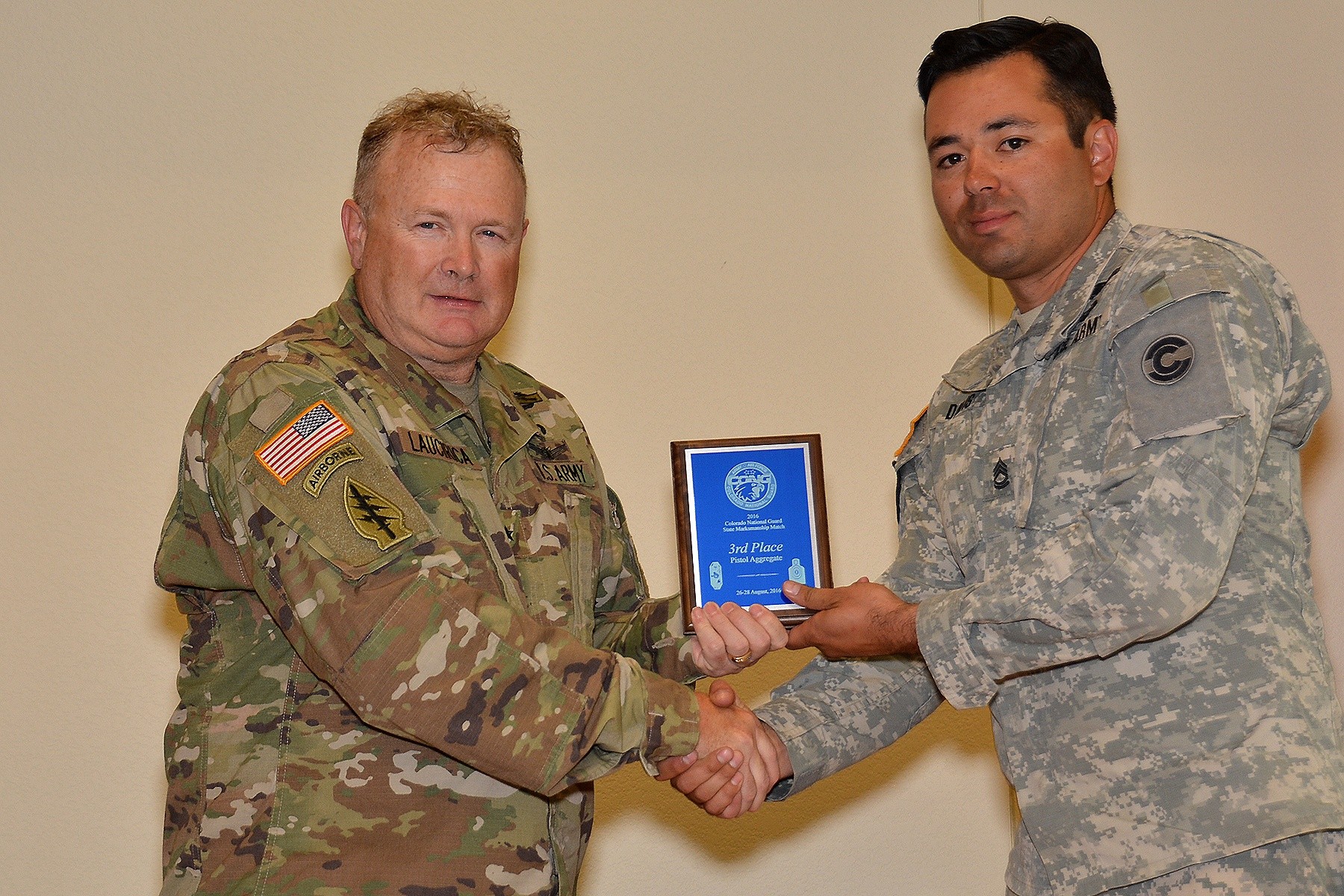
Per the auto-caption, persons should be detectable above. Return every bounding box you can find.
[656,17,1344,896]
[151,88,784,896]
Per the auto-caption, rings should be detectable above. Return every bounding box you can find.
[727,651,752,663]
